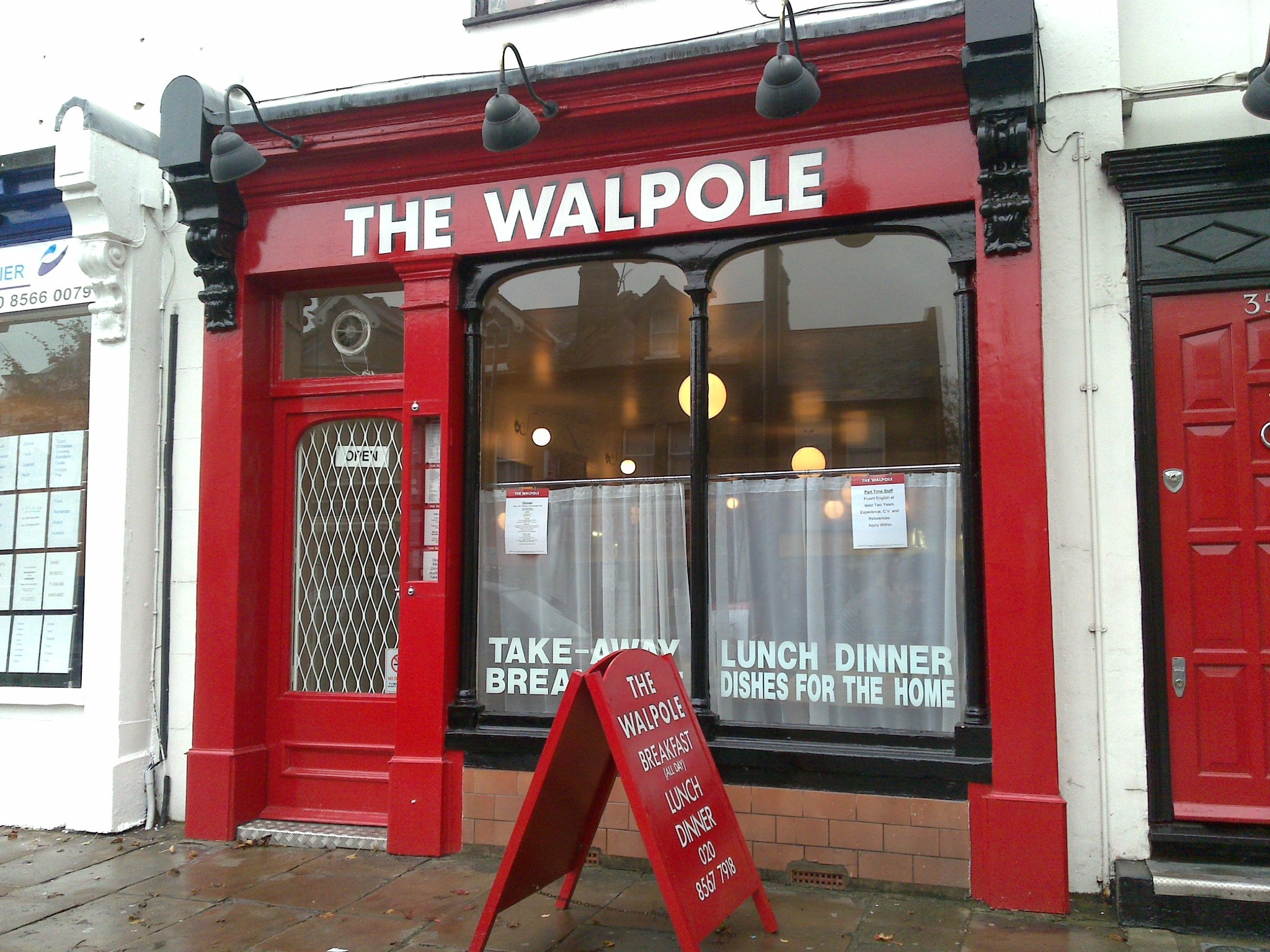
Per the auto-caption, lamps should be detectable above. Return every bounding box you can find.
[482,42,559,151]
[515,413,551,447]
[1242,24,1270,120]
[210,85,303,183]
[605,447,636,475]
[756,0,821,118]
